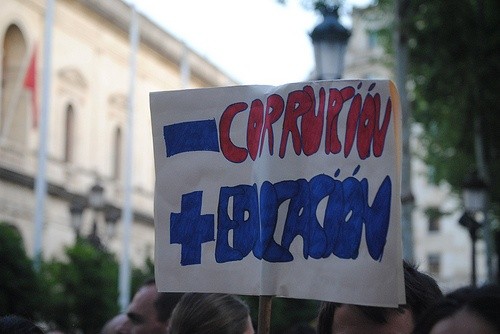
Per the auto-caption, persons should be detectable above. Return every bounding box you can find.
[167,291,255,334]
[424,283,500,334]
[105,279,185,334]
[0,315,47,334]
[320,260,443,334]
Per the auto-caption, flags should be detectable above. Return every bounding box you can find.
[24,51,37,129]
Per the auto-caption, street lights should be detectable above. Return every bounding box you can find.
[461,169,489,285]
